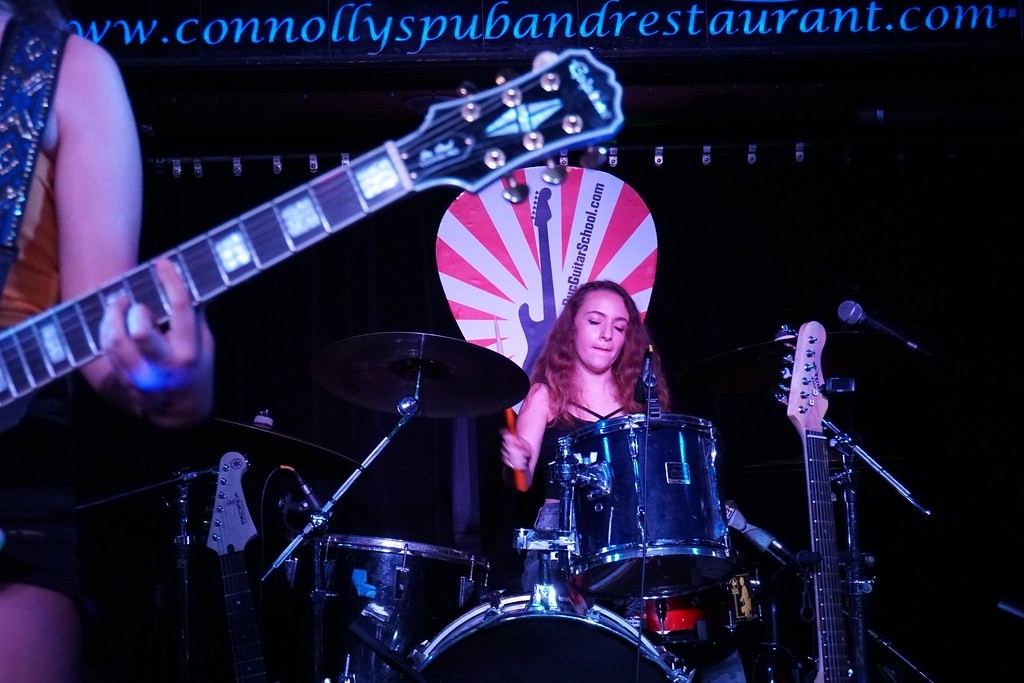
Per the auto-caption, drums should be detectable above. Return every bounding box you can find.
[640,571,764,646]
[555,411,736,602]
[405,590,695,683]
[311,533,491,683]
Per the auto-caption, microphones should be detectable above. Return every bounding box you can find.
[838,300,919,351]
[725,507,792,556]
[634,346,658,403]
[296,472,329,532]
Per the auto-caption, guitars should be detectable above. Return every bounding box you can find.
[203,451,270,683]
[774,320,851,683]
[1,47,628,410]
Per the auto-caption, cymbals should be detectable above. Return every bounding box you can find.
[308,331,530,420]
[206,415,362,469]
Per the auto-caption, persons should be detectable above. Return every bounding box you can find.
[501,280,669,593]
[0,0,216,683]
